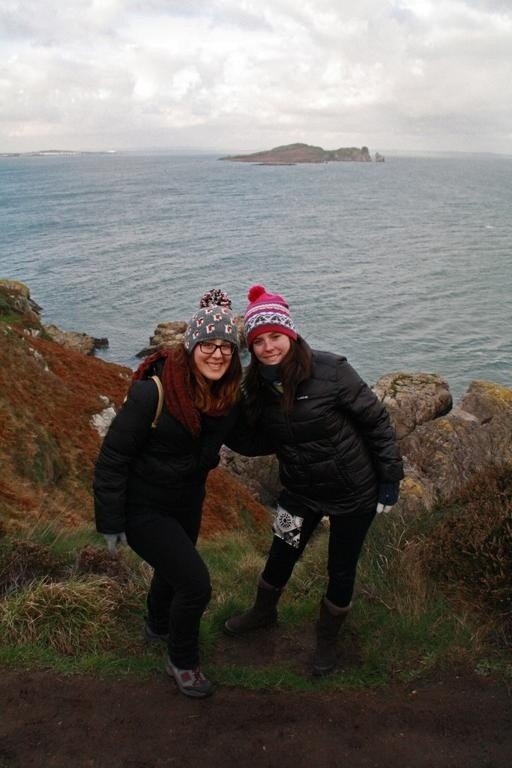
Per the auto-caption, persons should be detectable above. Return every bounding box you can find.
[217,283,403,677]
[92,287,275,698]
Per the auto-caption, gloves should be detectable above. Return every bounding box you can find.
[103,532,128,557]
[373,480,400,515]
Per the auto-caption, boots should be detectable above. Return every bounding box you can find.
[311,595,353,675]
[224,570,286,636]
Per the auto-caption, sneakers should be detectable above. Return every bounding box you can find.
[165,656,214,699]
[142,614,170,640]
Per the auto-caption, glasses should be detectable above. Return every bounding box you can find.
[196,339,236,356]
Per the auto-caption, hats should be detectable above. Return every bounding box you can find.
[240,283,300,344]
[184,287,239,355]
